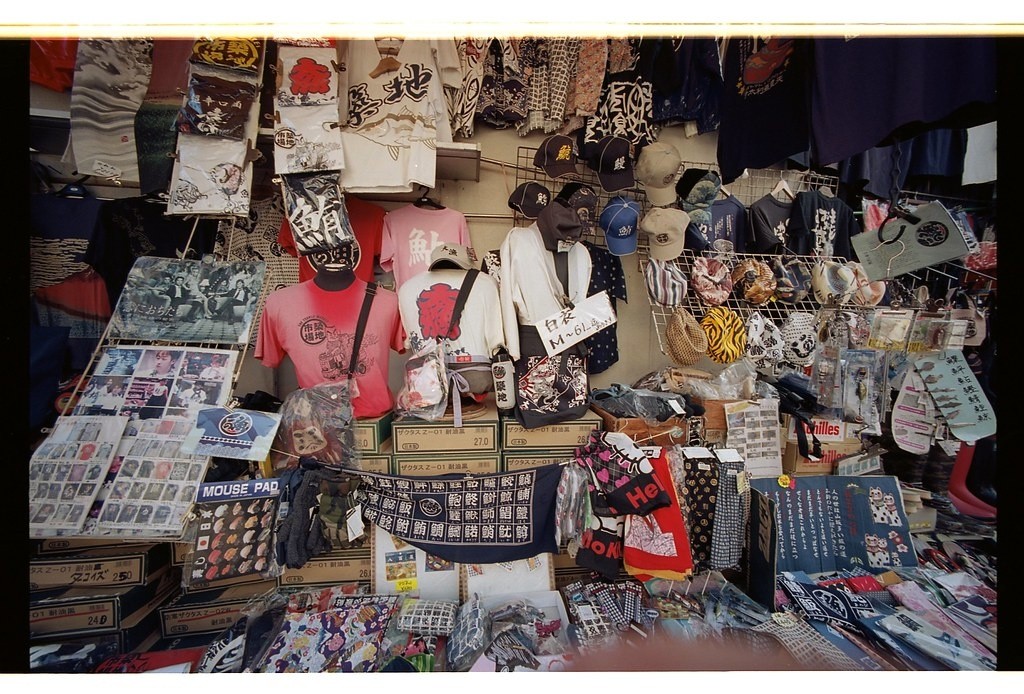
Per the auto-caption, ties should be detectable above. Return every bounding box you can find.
[234,290,241,299]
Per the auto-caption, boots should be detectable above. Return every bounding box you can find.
[903,437,998,536]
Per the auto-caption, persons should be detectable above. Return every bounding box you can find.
[29,422,113,536]
[74,349,228,420]
[100,420,207,527]
[124,263,257,325]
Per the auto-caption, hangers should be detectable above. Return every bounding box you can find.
[46,169,174,202]
[368,36,401,79]
[824,238,925,311]
[716,162,835,198]
[558,417,681,466]
[412,184,446,211]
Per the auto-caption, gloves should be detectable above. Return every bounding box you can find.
[275,456,364,569]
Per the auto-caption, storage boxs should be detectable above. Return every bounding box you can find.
[31,401,938,643]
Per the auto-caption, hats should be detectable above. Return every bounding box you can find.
[646,258,886,369]
[427,243,479,272]
[508,133,722,261]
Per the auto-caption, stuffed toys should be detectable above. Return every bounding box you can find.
[274,415,342,468]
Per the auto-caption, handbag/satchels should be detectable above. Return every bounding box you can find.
[396,598,458,637]
[445,592,485,671]
[945,308,986,346]
[271,379,352,468]
[452,365,493,394]
[513,355,591,429]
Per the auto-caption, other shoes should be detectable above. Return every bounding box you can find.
[202,309,222,321]
[227,317,235,325]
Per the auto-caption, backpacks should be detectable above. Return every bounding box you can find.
[393,339,449,422]
